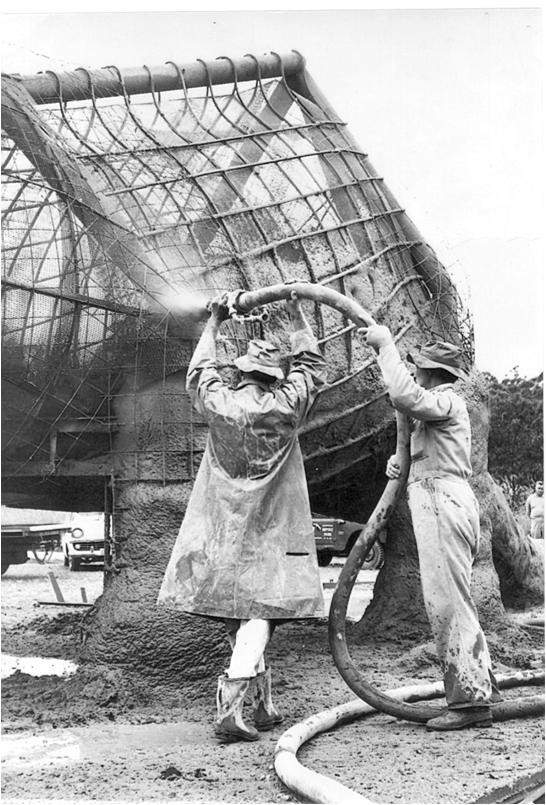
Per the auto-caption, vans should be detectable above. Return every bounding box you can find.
[1,505,112,576]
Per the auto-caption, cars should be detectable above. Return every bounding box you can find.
[311,511,388,572]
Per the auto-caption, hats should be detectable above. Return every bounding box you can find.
[407,341,466,381]
[234,339,284,379]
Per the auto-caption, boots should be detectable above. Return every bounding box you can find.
[253,668,286,732]
[215,677,260,743]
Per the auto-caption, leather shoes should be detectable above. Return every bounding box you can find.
[425,707,492,731]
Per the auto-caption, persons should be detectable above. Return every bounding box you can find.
[158,278,328,740]
[357,326,505,730]
[526,481,544,539]
